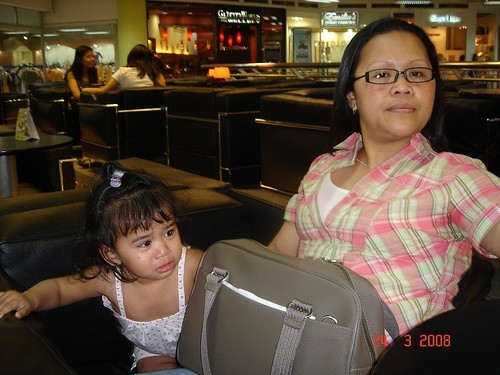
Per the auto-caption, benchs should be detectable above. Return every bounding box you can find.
[0,80,499,375]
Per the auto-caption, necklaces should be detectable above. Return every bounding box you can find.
[356,158,372,168]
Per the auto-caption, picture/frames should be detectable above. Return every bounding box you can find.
[293,28,312,63]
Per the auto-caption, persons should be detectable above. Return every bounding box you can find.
[81,44,166,95]
[0,162,203,371]
[65,45,103,118]
[267,18,500,344]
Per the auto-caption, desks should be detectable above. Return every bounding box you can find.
[0,134,73,154]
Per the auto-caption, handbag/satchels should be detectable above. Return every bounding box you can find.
[175,239,399,374]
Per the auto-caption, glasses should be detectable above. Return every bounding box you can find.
[353,67,438,84]
